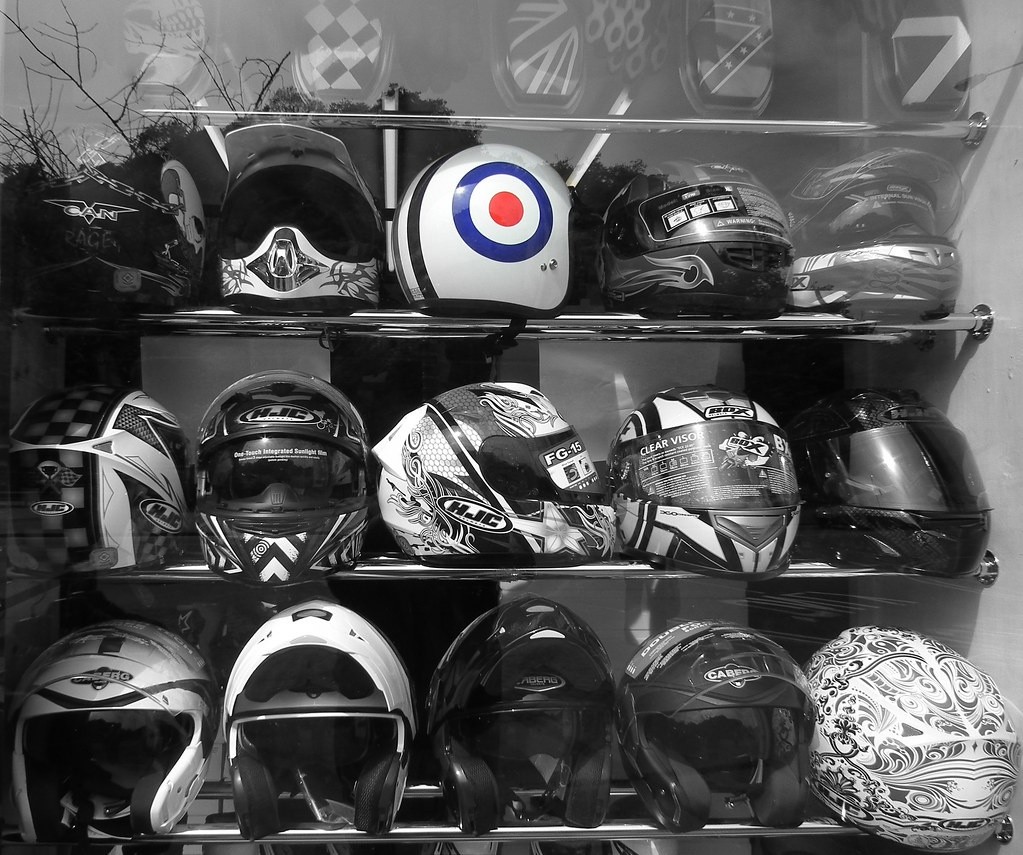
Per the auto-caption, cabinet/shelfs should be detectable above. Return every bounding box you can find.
[61,102,1015,842]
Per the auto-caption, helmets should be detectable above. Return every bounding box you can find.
[222,599,418,839]
[370,383,618,567]
[214,121,384,316]
[390,141,580,319]
[786,384,994,577]
[801,624,1022,852]
[192,370,373,589]
[17,136,200,313]
[594,159,791,318]
[8,619,220,844]
[783,147,963,317]
[424,597,616,838]
[605,382,805,581]
[617,619,817,833]
[2,380,194,575]
